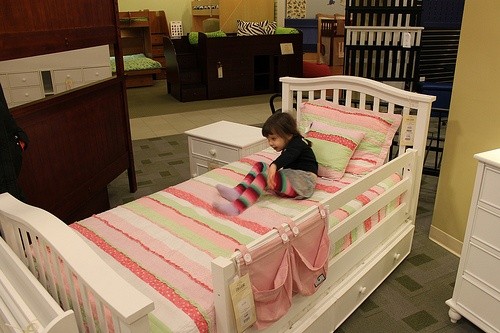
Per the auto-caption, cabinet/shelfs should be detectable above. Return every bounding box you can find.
[445,148,500,333]
[162,27,305,103]
[0,44,112,108]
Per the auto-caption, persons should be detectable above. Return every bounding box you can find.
[0,81,29,202]
[213,112,318,216]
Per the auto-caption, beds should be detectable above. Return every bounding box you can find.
[0,74,437,333]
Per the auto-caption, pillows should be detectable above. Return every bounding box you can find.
[236,20,277,35]
[295,98,403,182]
[276,26,300,35]
[187,30,226,45]
[304,120,365,179]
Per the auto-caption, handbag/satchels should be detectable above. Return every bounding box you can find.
[284,203,331,296]
[234,224,294,330]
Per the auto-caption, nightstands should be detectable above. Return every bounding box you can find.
[184,120,271,179]
